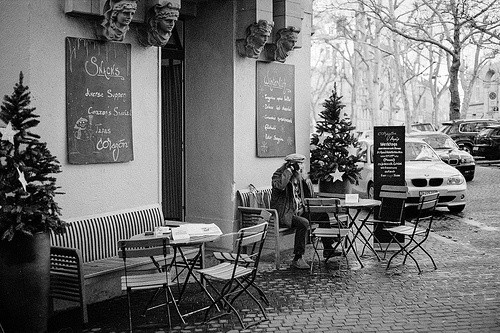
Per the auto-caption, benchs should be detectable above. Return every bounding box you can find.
[50,203,199,325]
[238,179,350,269]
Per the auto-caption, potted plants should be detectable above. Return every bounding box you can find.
[310,81,367,195]
[0,71,70,333]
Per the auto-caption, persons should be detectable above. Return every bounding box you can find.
[242,20,273,59]
[269,26,300,62]
[99,0,137,41]
[139,0,181,47]
[270,154,344,269]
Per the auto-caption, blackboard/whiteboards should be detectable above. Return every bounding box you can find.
[256,60,297,158]
[65,36,134,165]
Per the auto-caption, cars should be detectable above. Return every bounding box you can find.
[346,123,475,216]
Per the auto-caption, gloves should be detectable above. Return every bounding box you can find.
[289,163,300,170]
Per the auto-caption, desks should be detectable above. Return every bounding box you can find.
[325,199,382,268]
[129,232,222,326]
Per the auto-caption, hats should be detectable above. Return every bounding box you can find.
[284,154,306,163]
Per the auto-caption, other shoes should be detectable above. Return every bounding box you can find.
[292,258,310,269]
[323,249,347,258]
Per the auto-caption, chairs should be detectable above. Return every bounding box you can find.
[196,222,270,330]
[382,192,440,274]
[213,209,272,306]
[304,196,351,277]
[117,236,178,333]
[358,184,409,260]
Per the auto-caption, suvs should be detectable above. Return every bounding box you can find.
[438,118,500,164]
